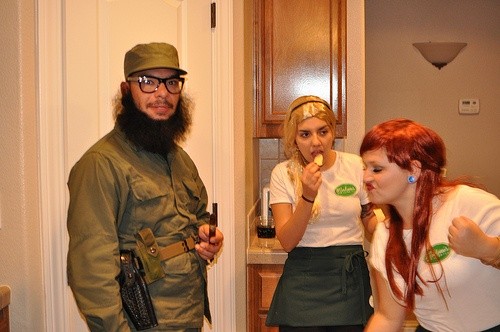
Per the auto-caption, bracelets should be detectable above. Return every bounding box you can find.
[301,195,315,204]
[480,235,500,267]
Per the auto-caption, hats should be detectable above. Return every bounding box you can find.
[124,43,187,78]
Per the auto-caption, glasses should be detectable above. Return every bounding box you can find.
[127,76,185,94]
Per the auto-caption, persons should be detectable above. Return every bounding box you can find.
[67,43,224,332]
[265,95,382,332]
[360,119,500,332]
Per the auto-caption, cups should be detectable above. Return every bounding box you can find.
[255,215,275,248]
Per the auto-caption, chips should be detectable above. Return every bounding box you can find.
[314,153,323,166]
